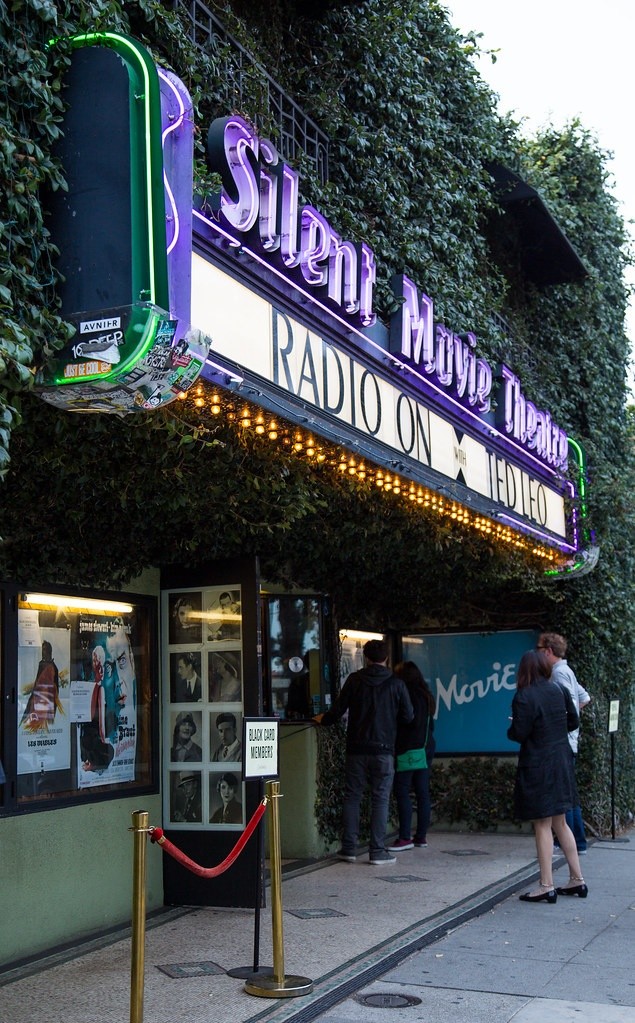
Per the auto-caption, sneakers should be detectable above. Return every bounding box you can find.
[369,852,396,865]
[387,839,414,851]
[334,849,356,861]
[411,834,428,847]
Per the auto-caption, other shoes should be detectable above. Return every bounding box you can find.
[577,850,587,854]
[553,846,565,857]
[553,837,560,846]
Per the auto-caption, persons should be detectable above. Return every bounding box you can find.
[208,652,241,702]
[311,640,414,867]
[169,653,202,703]
[535,633,592,856]
[170,711,203,762]
[386,661,435,852]
[284,650,328,722]
[211,713,242,762]
[23,623,138,774]
[169,596,202,643]
[506,651,588,904]
[207,592,240,641]
[170,770,202,823]
[209,772,242,825]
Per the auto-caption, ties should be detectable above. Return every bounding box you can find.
[188,682,191,692]
[224,748,228,757]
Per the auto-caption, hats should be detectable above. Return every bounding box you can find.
[174,712,196,736]
[212,653,240,678]
[177,771,199,788]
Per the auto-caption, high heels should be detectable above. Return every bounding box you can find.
[518,889,557,903]
[555,884,588,898]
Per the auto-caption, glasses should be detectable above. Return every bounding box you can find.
[535,645,547,652]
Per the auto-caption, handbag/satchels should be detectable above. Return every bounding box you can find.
[396,748,428,772]
